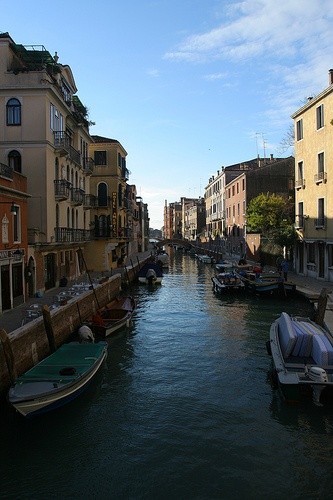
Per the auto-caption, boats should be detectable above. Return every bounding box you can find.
[4,338,108,416]
[233,268,280,286]
[156,243,194,263]
[269,312,333,383]
[211,272,245,293]
[138,262,163,288]
[196,253,216,263]
[233,258,252,268]
[214,258,232,271]
[80,293,136,336]
